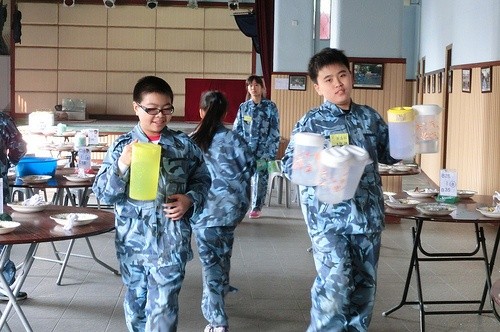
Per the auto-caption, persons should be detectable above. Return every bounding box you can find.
[0,112,27,301]
[93,76,212,332]
[280,48,402,332]
[232,75,280,217]
[188,91,257,332]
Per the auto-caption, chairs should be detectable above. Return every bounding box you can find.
[265,160,301,210]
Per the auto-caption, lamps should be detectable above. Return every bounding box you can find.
[62,0,239,12]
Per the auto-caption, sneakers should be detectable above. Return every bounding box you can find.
[0,291,27,300]
[204,324,228,332]
[249,210,262,218]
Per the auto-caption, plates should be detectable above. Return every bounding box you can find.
[19,174,52,184]
[62,173,95,182]
[384,198,420,209]
[457,190,476,199]
[7,200,51,214]
[403,189,439,198]
[476,206,500,219]
[0,220,21,234]
[378,163,417,171]
[49,212,99,226]
[416,203,457,216]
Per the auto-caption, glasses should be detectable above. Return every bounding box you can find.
[137,102,174,115]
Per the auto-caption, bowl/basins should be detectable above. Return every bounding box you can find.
[15,156,60,177]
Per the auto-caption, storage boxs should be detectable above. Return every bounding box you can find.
[60,110,87,120]
[29,112,54,126]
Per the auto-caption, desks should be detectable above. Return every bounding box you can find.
[383,194,500,332]
[378,162,422,176]
[0,126,122,332]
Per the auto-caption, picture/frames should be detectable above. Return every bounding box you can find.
[352,62,385,90]
[461,68,472,93]
[480,64,492,93]
[416,70,453,94]
[288,75,307,91]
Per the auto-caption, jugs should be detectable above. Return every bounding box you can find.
[78,146,91,172]
[129,142,161,201]
[387,104,442,160]
[291,131,374,205]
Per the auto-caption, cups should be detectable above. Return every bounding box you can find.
[87,129,99,146]
